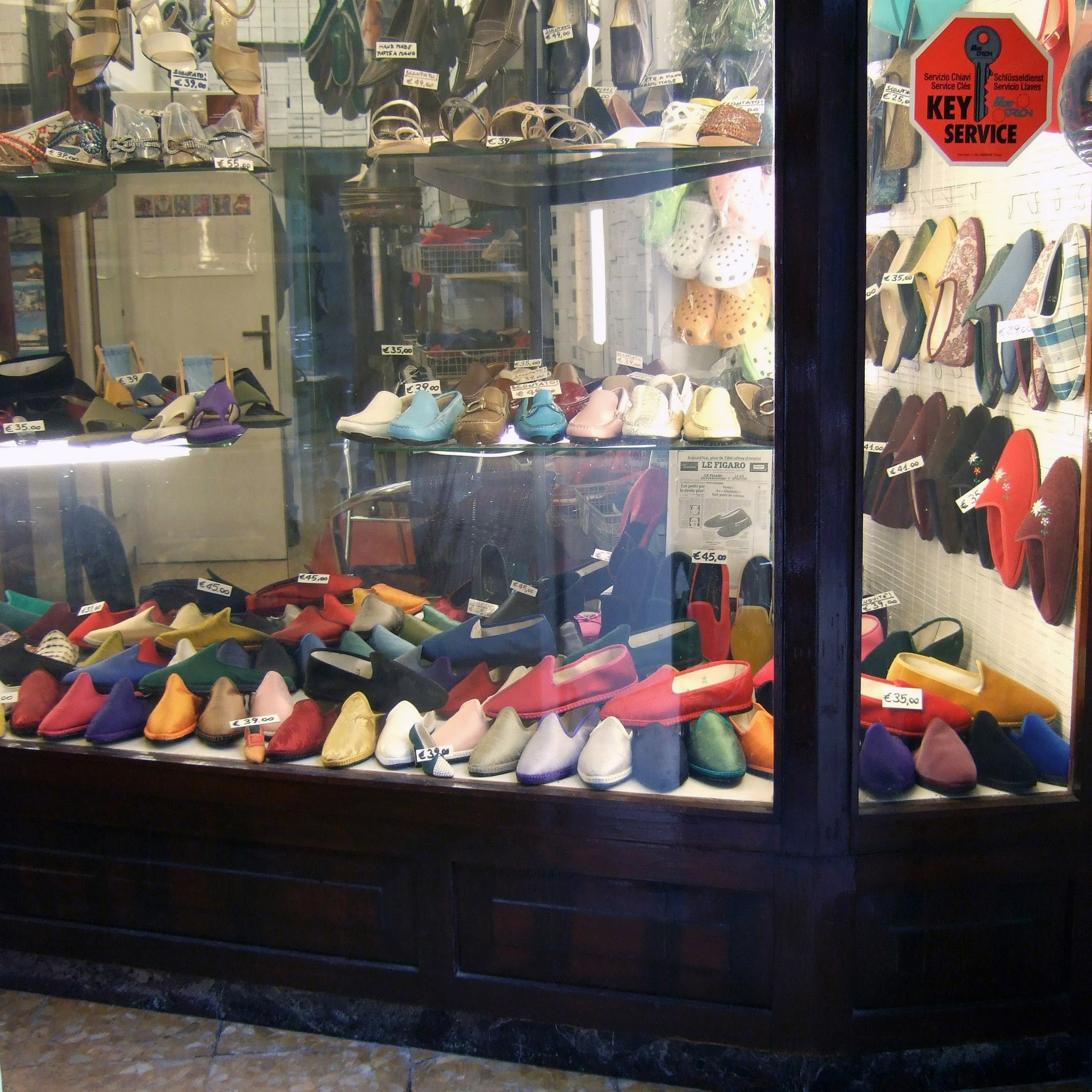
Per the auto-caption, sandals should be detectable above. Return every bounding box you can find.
[2,0,763,166]
[663,168,774,348]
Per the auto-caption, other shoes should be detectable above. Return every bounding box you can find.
[417,326,529,358]
[0,346,1073,800]
[864,217,1091,412]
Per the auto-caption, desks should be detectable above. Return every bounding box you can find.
[0,414,292,564]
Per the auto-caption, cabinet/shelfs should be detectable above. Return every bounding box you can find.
[360,145,775,454]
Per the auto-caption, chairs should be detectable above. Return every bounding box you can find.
[328,468,557,610]
